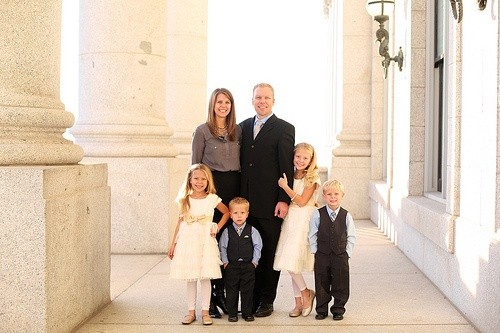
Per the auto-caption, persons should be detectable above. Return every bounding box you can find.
[219,198,263,323]
[237,83,295,317]
[307,180,354,321]
[167,163,230,325]
[272,143,322,317]
[191,87,242,319]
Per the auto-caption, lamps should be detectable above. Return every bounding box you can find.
[367,0,403,79]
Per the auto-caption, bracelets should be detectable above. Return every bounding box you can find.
[291,192,299,201]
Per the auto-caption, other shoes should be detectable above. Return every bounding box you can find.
[202,314,212,325]
[290,306,302,317]
[302,289,316,317]
[182,313,196,324]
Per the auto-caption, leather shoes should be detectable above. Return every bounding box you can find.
[227,312,238,322]
[315,313,329,320]
[210,298,221,318]
[255,303,273,317]
[213,295,230,315]
[243,311,254,321]
[333,314,343,320]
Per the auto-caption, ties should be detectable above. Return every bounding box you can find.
[253,120,263,139]
[330,212,336,222]
[237,227,242,236]
